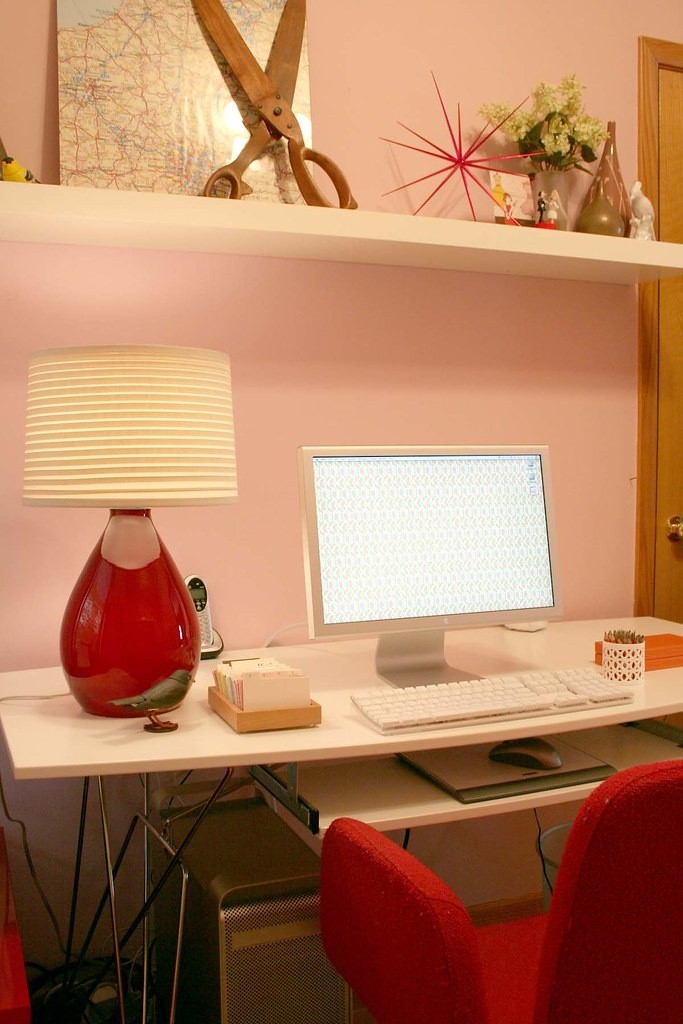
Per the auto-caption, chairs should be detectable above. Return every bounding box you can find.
[317,758,683,1024]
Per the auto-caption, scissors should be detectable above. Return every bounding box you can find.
[193,0,358,209]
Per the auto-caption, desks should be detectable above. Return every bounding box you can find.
[1,616,683,1023]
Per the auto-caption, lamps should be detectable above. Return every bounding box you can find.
[21,350,237,718]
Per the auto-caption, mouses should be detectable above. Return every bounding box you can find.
[489,737,563,769]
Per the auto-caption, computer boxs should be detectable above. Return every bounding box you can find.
[150,776,354,1024]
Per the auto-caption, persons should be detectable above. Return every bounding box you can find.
[536,190,558,224]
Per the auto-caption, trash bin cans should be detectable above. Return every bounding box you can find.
[537,820,577,911]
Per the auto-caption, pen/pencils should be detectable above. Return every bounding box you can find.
[602,630,645,685]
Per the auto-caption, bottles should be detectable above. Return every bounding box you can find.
[580,122,632,238]
[575,179,625,237]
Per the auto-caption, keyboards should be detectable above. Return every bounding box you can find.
[351,666,636,736]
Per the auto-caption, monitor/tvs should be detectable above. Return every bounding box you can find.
[298,443,563,686]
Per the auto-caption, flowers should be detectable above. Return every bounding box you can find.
[476,72,612,176]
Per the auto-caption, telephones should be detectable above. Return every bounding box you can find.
[183,574,225,660]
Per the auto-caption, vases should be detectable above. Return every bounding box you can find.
[572,120,634,240]
[535,172,568,232]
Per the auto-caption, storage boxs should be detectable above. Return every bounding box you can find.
[594,633,683,672]
[207,687,322,732]
